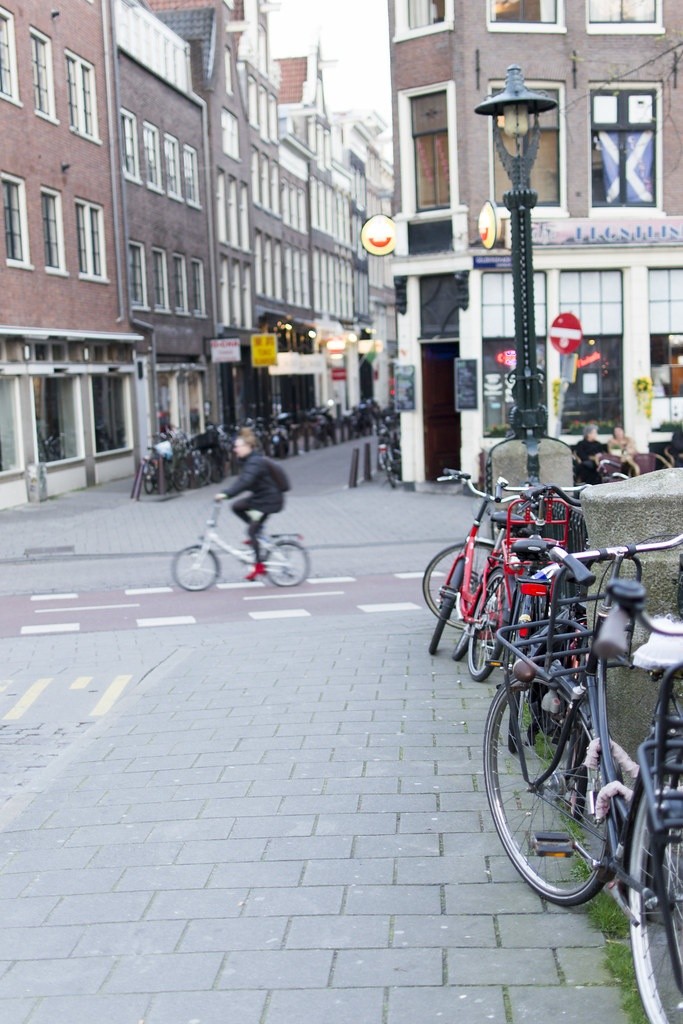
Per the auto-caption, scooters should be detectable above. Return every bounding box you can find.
[139,392,383,494]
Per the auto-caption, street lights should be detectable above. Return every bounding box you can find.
[475,61,561,436]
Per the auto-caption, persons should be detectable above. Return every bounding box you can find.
[669,419,683,468]
[211,427,285,581]
[576,423,637,486]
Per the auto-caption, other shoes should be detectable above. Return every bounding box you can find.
[244,563,268,581]
[243,539,252,544]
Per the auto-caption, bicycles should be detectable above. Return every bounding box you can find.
[170,496,311,592]
[418,461,591,822]
[376,414,403,488]
[483,532,683,1024]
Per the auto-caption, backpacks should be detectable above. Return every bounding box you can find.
[254,453,290,492]
